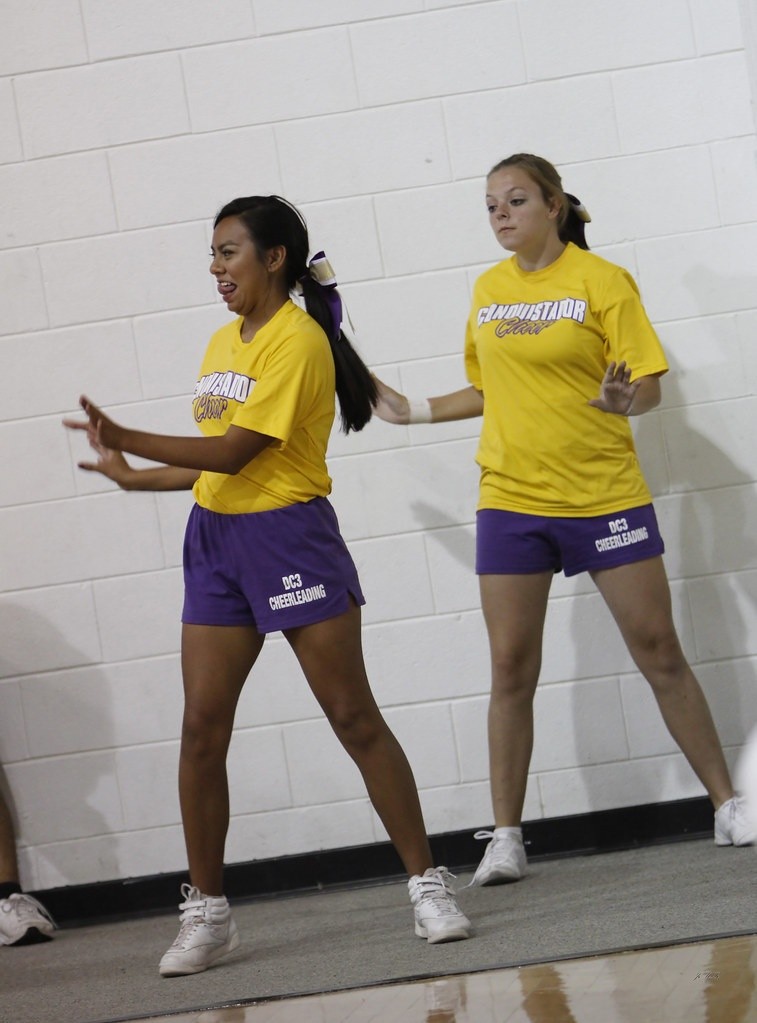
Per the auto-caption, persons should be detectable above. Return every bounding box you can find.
[60,196,473,978]
[366,152,757,885]
[0,764,56,948]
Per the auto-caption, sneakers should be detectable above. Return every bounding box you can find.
[462,827,528,890]
[408,865,472,943]
[159,882,240,974]
[0,892,59,946]
[714,798,757,845]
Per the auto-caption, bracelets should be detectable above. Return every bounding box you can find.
[408,398,432,423]
